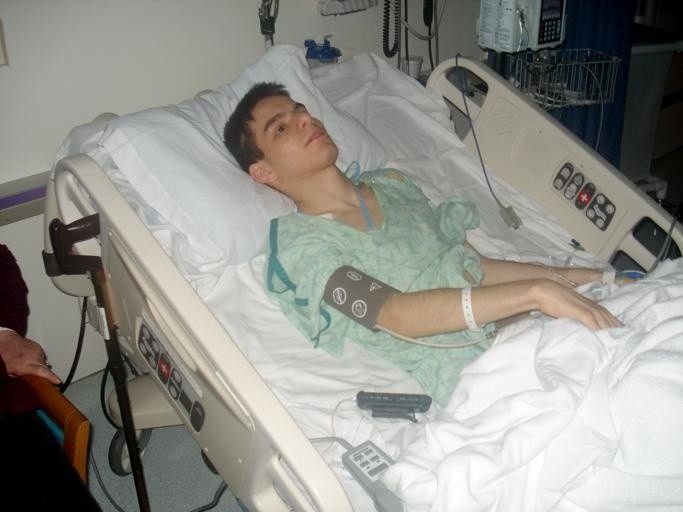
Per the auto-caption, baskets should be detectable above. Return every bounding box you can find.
[508,46,621,111]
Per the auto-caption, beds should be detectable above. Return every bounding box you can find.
[42,51,683,512]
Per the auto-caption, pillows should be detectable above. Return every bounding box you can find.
[97,43,388,274]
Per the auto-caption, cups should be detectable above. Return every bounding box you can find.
[401,56,423,81]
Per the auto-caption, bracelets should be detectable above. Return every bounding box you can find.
[599,265,618,290]
[458,284,490,336]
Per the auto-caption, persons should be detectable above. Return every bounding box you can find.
[0,246,102,512]
[227,82,643,416]
